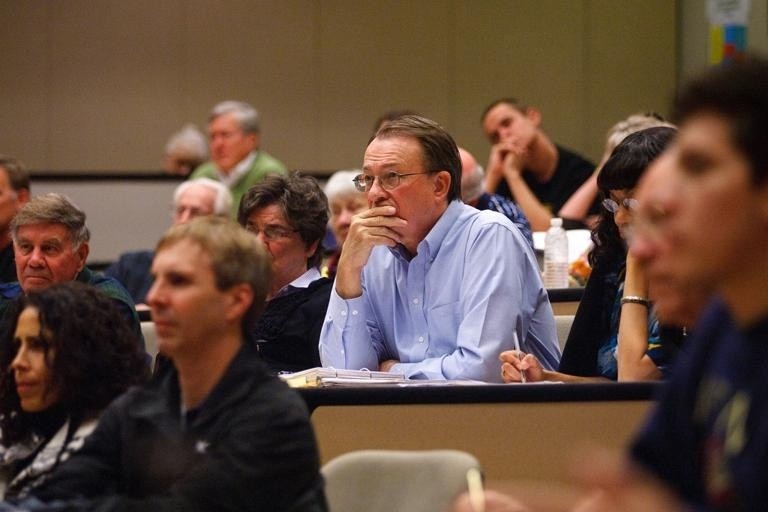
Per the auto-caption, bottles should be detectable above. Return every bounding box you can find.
[542,218,570,290]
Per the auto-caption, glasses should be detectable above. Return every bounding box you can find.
[601,198,639,214]
[353,171,438,192]
[246,224,301,240]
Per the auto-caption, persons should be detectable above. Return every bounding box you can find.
[499,127,686,382]
[451,57,767,511]
[167,101,284,223]
[459,98,664,248]
[17,223,329,512]
[4,190,146,364]
[237,173,335,372]
[319,111,562,383]
[1,288,153,512]
[0,156,36,277]
[324,169,368,241]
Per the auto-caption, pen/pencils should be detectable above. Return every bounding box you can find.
[466,467,486,512]
[511,331,529,384]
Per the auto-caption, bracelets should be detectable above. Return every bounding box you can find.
[621,296,648,305]
